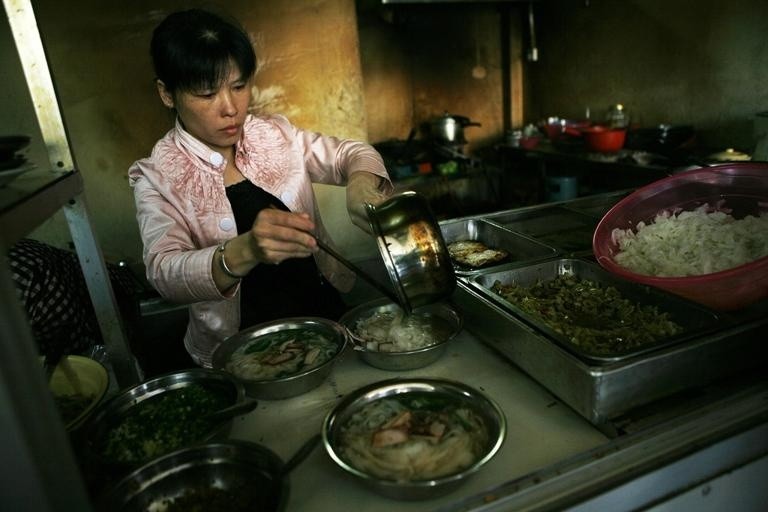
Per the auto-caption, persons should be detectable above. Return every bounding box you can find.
[6,239,142,356]
[127,8,395,371]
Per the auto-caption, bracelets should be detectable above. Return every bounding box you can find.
[220,241,245,278]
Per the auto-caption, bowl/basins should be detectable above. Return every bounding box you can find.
[544,121,627,152]
[520,136,540,151]
[322,376,507,502]
[210,315,351,402]
[91,439,291,511]
[39,353,110,434]
[86,366,247,476]
[338,294,464,371]
[593,162,768,313]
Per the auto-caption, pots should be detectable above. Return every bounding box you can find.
[429,110,481,147]
[363,190,457,319]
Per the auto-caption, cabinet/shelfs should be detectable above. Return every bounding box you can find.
[1,1,142,484]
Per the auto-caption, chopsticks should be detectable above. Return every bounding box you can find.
[267,206,406,308]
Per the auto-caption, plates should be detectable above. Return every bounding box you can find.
[1,133,38,188]
